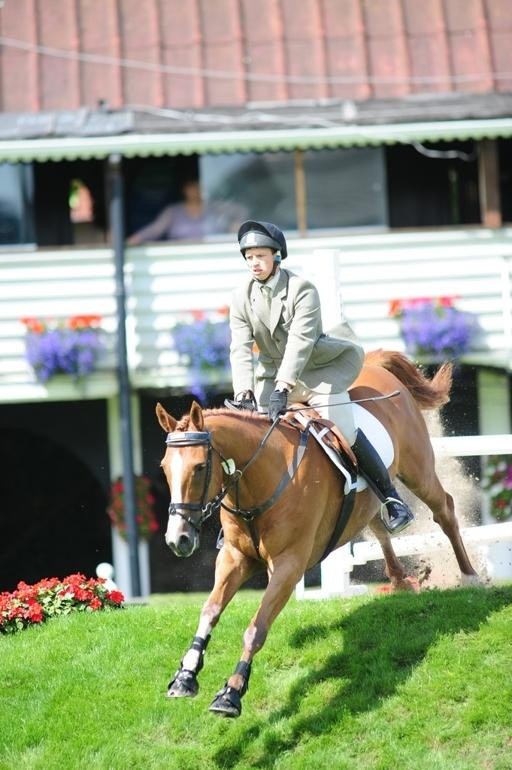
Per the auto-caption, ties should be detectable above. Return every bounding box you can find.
[262,287,271,306]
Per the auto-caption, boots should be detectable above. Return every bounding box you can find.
[350,427,414,531]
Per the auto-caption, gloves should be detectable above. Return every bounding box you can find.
[268,389,287,423]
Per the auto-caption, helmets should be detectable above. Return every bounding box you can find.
[238,220,287,260]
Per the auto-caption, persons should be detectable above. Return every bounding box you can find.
[228,219,417,533]
[123,175,225,249]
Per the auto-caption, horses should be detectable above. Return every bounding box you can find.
[155,349,484,718]
